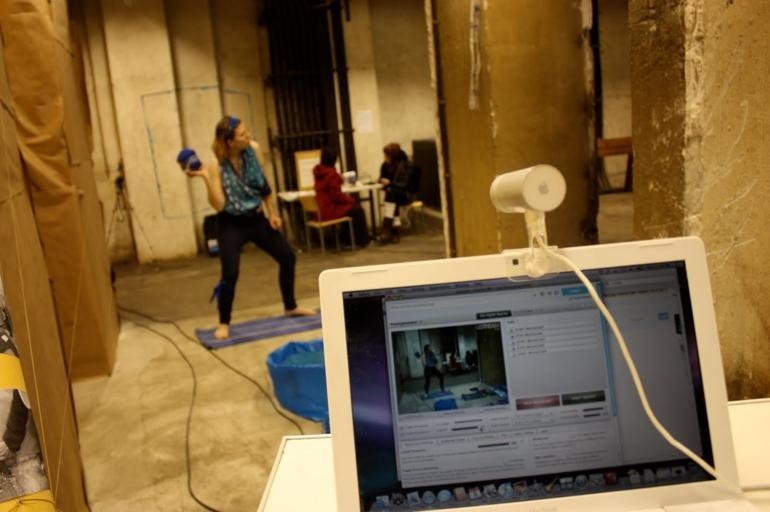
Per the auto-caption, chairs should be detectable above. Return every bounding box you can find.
[277,175,423,257]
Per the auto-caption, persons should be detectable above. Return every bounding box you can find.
[312,142,373,250]
[185,114,316,340]
[413,342,478,400]
[374,141,427,244]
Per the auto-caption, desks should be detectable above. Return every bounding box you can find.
[255,396,770,512]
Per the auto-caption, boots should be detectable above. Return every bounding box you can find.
[381,218,401,242]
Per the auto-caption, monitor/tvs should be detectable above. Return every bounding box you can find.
[316,234,763,512]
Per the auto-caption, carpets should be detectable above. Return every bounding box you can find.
[195,306,322,350]
